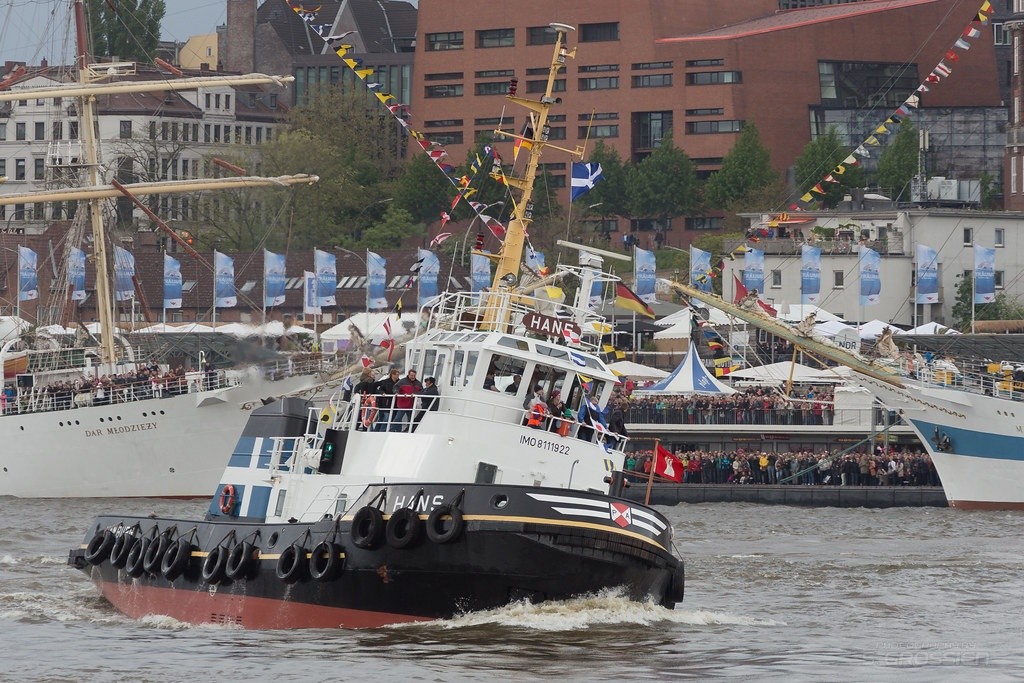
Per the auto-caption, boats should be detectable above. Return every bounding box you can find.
[0,0,355,498]
[66,22,685,628]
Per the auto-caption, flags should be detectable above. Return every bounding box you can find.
[65,244,87,302]
[470,246,491,311]
[634,243,658,305]
[614,279,656,319]
[313,249,338,307]
[570,160,604,202]
[654,443,684,484]
[303,269,324,316]
[366,252,389,310]
[418,247,442,312]
[17,245,39,303]
[690,0,996,312]
[262,249,287,309]
[162,254,183,309]
[520,250,625,440]
[113,245,137,304]
[213,250,237,307]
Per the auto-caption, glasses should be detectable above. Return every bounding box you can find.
[490,373,494,375]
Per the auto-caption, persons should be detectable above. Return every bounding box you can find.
[354,366,439,434]
[594,230,665,251]
[607,378,938,487]
[485,368,629,449]
[0,359,216,416]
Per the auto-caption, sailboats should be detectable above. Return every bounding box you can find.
[654,0,1023,513]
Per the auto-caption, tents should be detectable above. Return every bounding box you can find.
[628,336,742,397]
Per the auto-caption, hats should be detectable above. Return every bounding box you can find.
[552,390,560,398]
[534,385,543,392]
[424,377,435,384]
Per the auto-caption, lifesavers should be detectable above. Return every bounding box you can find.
[161,538,191,581]
[220,485,234,512]
[427,504,464,544]
[202,546,227,585]
[126,537,150,577]
[530,404,543,421]
[361,396,376,428]
[84,530,116,565]
[226,541,252,579]
[386,508,421,546]
[349,507,384,550]
[310,542,339,581]
[276,545,306,580]
[143,535,169,572]
[110,533,133,568]
[673,562,685,603]
